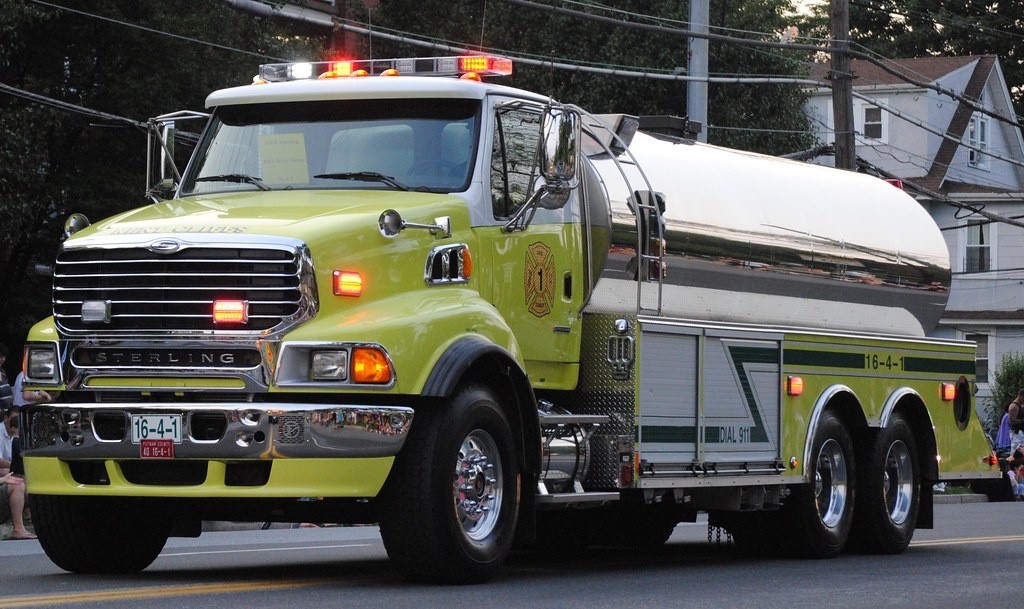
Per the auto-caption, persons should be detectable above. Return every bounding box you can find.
[0,344,53,539]
[309,407,408,435]
[994,388,1024,502]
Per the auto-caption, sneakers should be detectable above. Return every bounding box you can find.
[933,482,945,492]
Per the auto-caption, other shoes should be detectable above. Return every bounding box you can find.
[12,532,37,539]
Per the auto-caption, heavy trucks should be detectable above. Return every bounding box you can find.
[9,53,1005,587]
